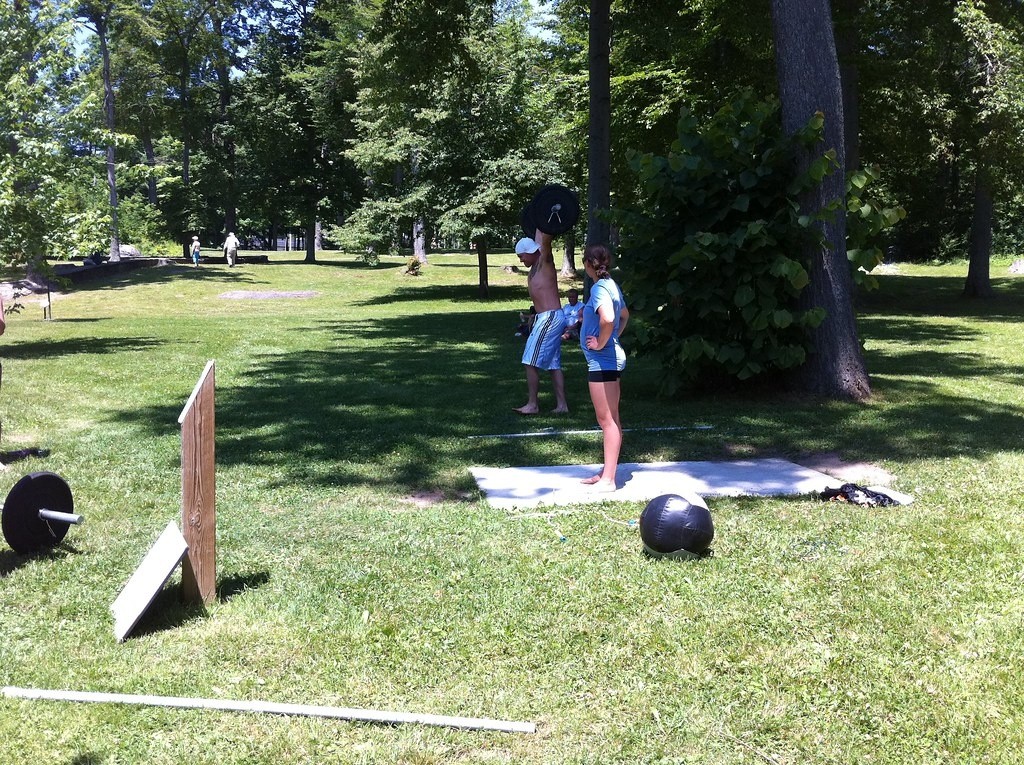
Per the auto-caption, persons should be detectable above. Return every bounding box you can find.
[579,242,628,494]
[190,236,201,268]
[561,307,583,341]
[519,306,537,333]
[0,293,6,440]
[562,289,585,329]
[222,232,241,267]
[512,226,571,415]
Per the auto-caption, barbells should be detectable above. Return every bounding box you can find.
[0,471,84,557]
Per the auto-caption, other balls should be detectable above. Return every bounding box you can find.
[640,492,714,558]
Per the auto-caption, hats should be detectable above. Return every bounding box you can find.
[514,237,540,255]
